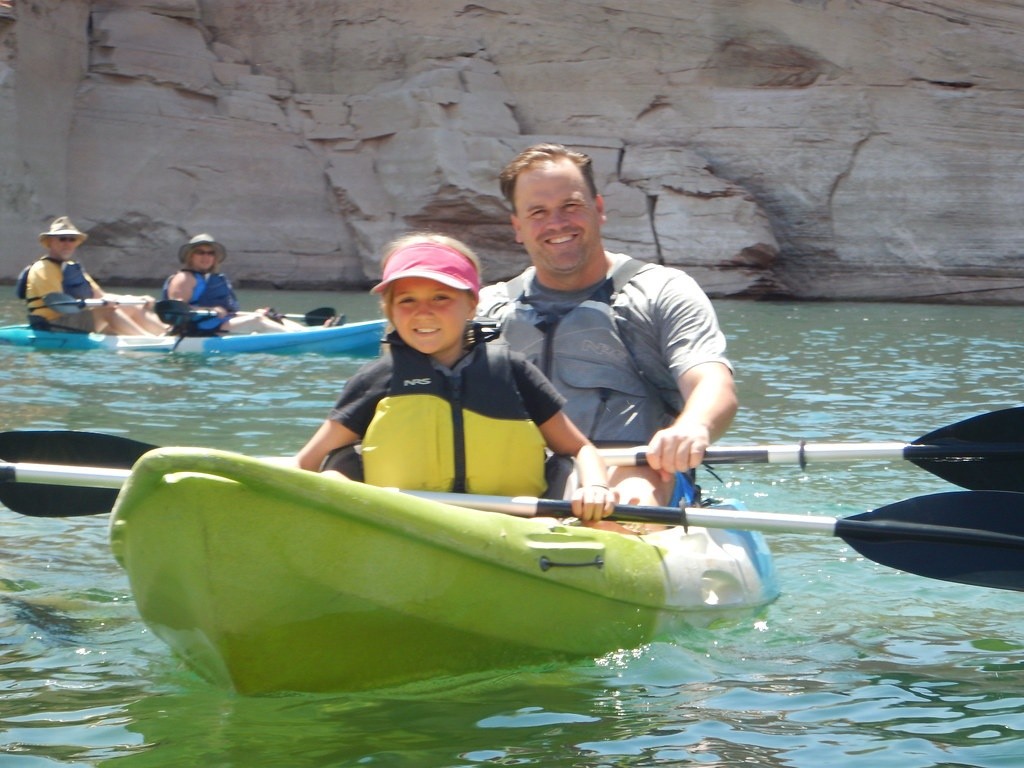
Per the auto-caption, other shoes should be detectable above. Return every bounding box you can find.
[325,314,346,327]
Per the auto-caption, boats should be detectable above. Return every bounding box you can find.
[1,312,394,360]
[107,445,784,700]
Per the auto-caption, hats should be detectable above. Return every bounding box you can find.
[39,216,88,247]
[370,244,475,293]
[178,234,226,267]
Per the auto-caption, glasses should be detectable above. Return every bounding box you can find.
[57,236,77,242]
[192,249,217,256]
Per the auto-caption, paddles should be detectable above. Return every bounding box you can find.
[1,404,1023,521]
[1,458,1023,592]
[154,298,338,327]
[42,292,160,317]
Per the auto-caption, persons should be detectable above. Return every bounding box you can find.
[26,216,170,337]
[293,143,739,535]
[166,233,305,335]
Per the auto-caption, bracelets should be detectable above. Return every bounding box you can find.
[592,484,609,491]
